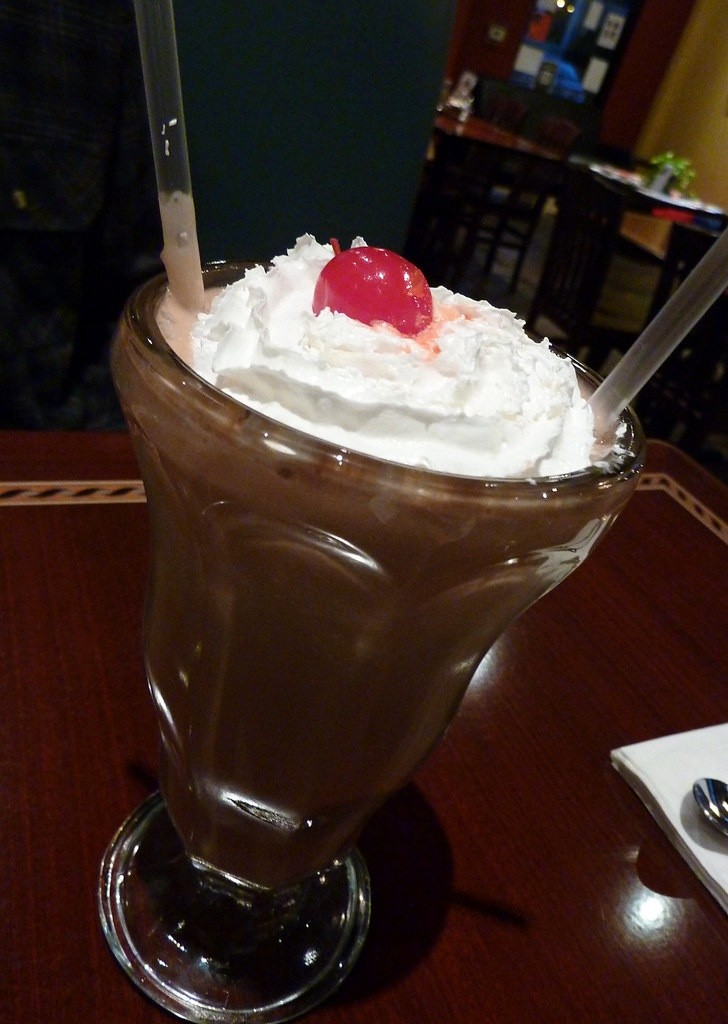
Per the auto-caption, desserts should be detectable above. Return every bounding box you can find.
[114,230,630,896]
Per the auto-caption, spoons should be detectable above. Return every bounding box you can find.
[693,778,728,838]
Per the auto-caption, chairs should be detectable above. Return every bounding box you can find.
[440,116,580,289]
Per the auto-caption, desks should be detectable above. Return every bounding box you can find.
[1,431,728,1024]
[408,114,561,272]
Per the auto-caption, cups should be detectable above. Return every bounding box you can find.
[93,259,643,1024]
